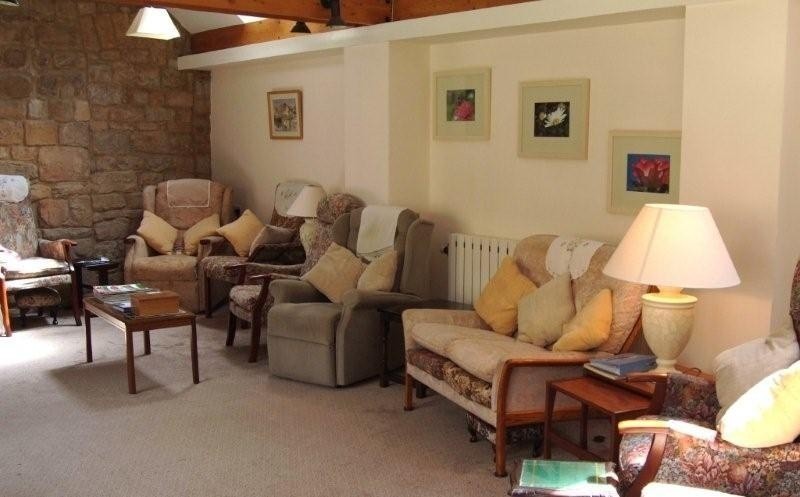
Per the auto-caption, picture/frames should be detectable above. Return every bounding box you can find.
[433,62,493,145]
[265,87,306,142]
[517,73,592,164]
[603,124,683,220]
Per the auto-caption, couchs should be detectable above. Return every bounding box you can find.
[396,233,665,482]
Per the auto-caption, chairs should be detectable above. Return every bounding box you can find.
[120,175,233,316]
[221,191,365,365]
[610,256,799,497]
[1,173,80,337]
[202,179,309,331]
[264,204,436,389]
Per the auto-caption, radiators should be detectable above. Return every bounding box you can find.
[438,228,516,307]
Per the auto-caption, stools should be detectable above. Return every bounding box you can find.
[15,287,64,328]
[463,408,545,462]
[503,457,624,497]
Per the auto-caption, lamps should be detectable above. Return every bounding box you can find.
[319,0,349,29]
[120,4,181,42]
[287,20,312,34]
[600,200,746,380]
[283,181,324,256]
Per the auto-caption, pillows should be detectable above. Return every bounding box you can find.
[136,206,180,257]
[546,285,616,356]
[472,256,538,340]
[300,241,366,306]
[217,207,265,257]
[248,222,297,261]
[710,321,799,425]
[718,360,799,446]
[513,268,579,350]
[357,247,401,294]
[183,211,220,258]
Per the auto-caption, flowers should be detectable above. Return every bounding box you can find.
[626,155,670,195]
[535,103,568,138]
[454,97,475,123]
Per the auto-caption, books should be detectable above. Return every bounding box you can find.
[93,282,146,305]
[583,352,658,381]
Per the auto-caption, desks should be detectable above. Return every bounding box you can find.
[72,256,120,319]
[82,294,202,395]
[539,362,714,477]
[376,298,475,401]
